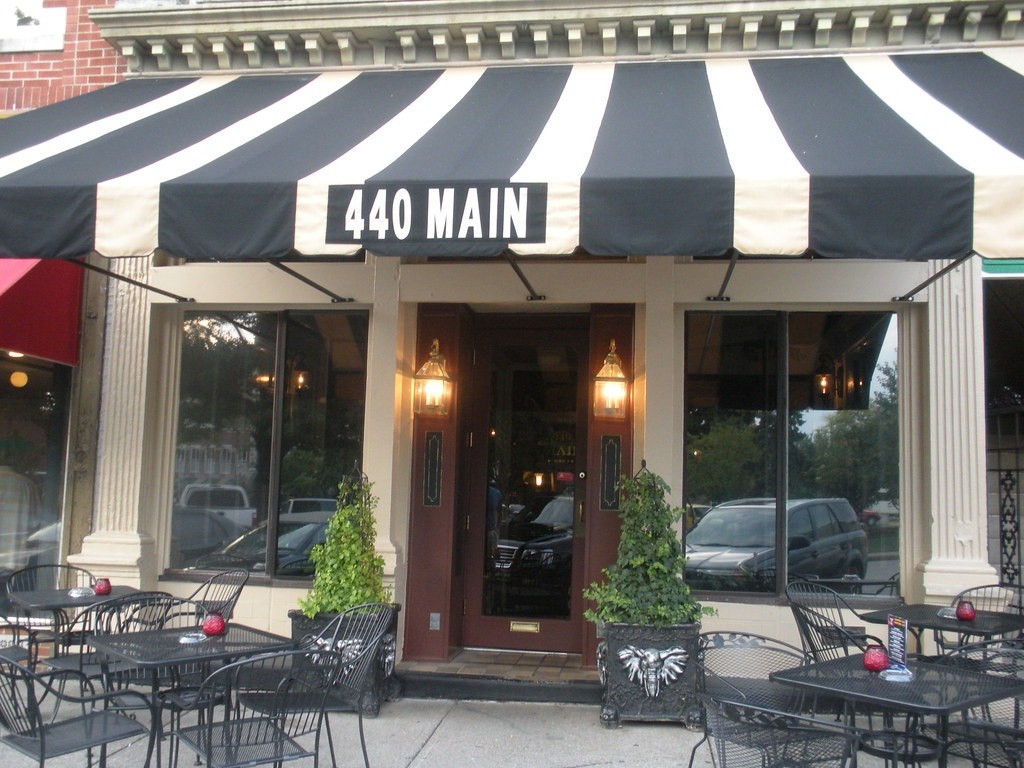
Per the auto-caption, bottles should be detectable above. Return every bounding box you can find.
[202,611,225,635]
[956,601,976,620]
[863,645,889,672]
[94,578,111,595]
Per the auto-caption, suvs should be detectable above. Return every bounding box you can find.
[683,497,867,594]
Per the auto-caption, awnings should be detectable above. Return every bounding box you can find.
[0,256,84,369]
[259,310,894,409]
[0,46,1024,300]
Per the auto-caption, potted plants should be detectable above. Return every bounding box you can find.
[287,459,401,720]
[580,459,719,733]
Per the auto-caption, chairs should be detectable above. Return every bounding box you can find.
[686,579,1024,768]
[0,563,394,768]
[874,572,900,594]
[739,564,769,592]
[195,553,250,570]
[281,558,315,577]
[757,568,811,592]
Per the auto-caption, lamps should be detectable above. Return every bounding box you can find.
[285,351,310,400]
[814,353,835,394]
[9,369,32,388]
[592,339,628,423]
[413,337,454,417]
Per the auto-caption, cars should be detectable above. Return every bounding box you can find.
[685,503,713,530]
[173,496,339,576]
[857,509,881,526]
[0,523,61,596]
[488,493,574,598]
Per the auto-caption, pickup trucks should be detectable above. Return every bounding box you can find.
[181,482,257,529]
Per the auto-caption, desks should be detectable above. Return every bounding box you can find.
[858,603,1024,733]
[85,622,297,768]
[5,586,162,727]
[209,566,305,576]
[768,653,1024,768]
[809,578,896,594]
